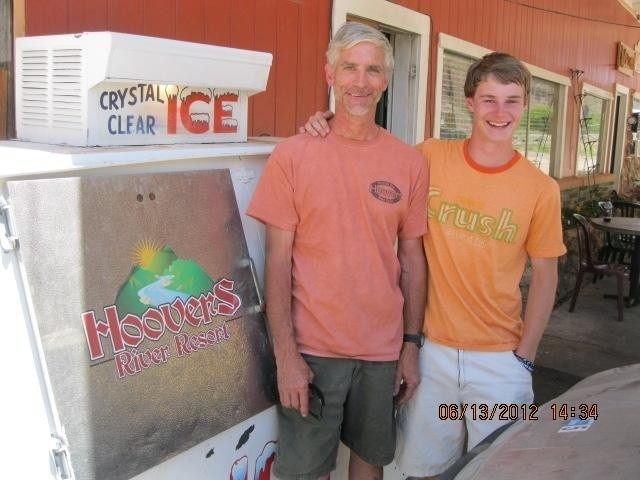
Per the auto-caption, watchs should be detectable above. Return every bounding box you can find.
[405,331,425,347]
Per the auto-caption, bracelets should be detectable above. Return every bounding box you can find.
[516,356,536,372]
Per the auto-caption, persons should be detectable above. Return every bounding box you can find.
[299,52,567,480]
[246,21,430,480]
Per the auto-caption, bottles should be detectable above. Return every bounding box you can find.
[603,200,613,222]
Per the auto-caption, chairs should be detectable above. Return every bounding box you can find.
[567,200,640,322]
